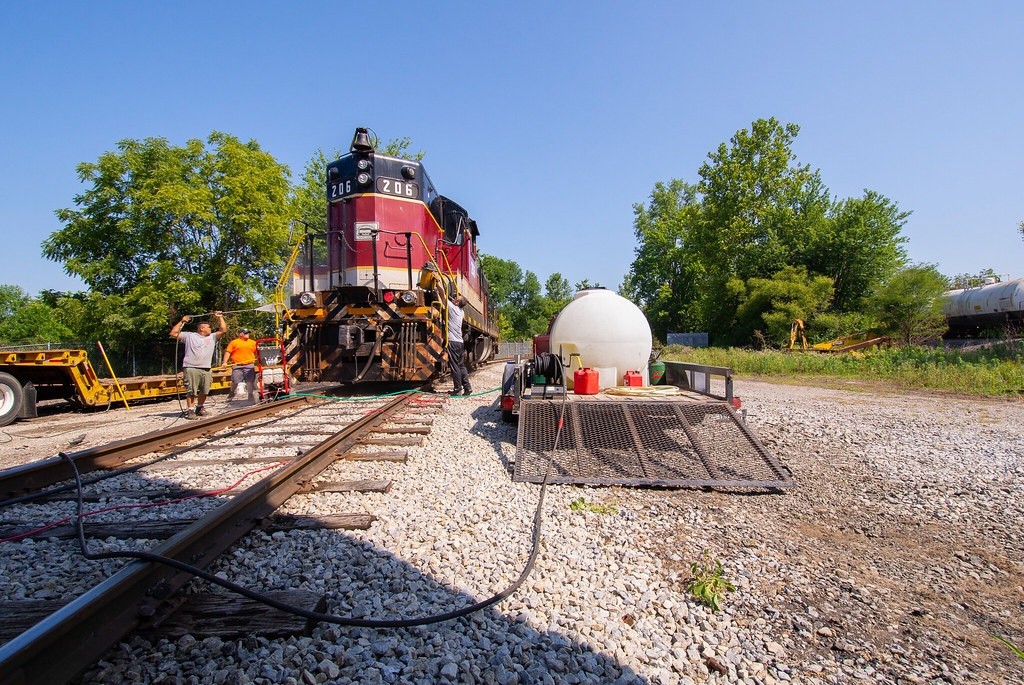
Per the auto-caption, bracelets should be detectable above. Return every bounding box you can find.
[436,279,440,282]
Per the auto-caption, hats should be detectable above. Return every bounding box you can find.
[238,328,251,334]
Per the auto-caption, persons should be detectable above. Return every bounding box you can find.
[170,312,226,420]
[221,329,258,402]
[432,272,472,395]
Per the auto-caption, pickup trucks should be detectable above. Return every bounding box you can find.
[533,313,557,357]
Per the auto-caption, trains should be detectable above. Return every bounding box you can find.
[275,126,499,391]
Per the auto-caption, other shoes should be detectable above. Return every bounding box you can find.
[227,391,236,402]
[448,390,461,396]
[463,388,472,395]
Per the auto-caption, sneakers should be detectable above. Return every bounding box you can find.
[185,409,200,421]
[196,405,212,416]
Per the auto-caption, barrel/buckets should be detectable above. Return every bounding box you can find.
[573,366,599,395]
[649,361,666,386]
[623,370,642,387]
[262,368,284,384]
